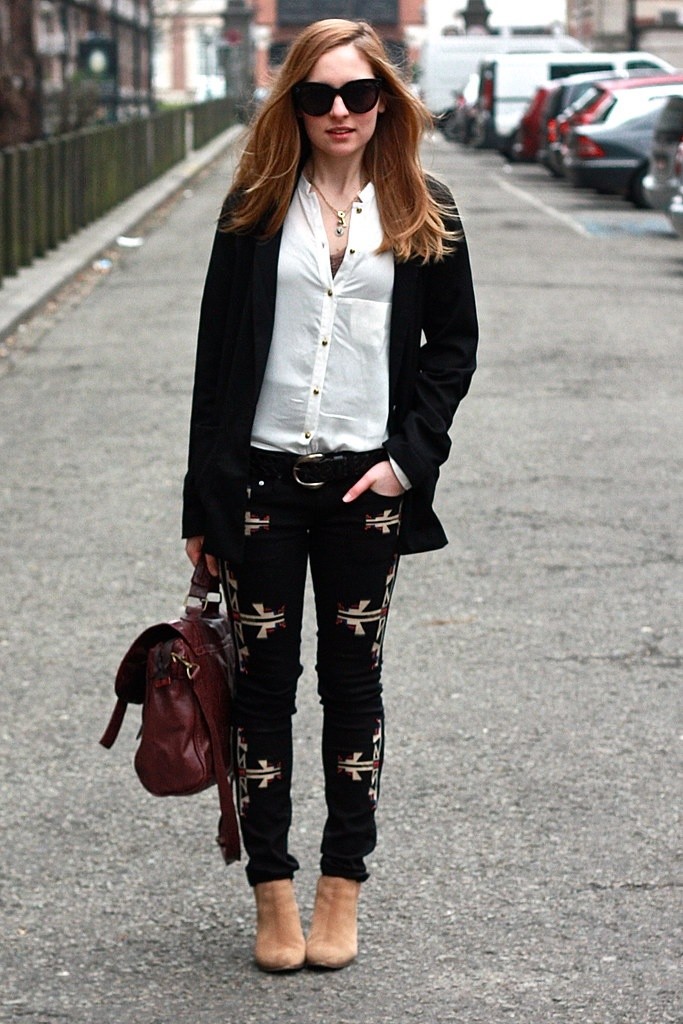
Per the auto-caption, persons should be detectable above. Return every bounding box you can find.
[183,21,480,970]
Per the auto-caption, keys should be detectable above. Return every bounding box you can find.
[337,210,347,227]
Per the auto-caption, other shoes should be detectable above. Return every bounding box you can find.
[254,878,306,974]
[305,874,359,969]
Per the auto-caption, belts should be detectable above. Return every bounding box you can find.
[248,447,395,490]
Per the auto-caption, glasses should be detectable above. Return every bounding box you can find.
[291,79,385,116]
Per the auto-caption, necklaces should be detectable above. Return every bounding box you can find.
[306,176,369,237]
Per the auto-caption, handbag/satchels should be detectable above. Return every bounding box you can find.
[99,556,241,867]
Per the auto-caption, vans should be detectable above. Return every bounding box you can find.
[416,31,588,139]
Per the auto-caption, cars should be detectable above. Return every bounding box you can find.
[447,49,683,237]
